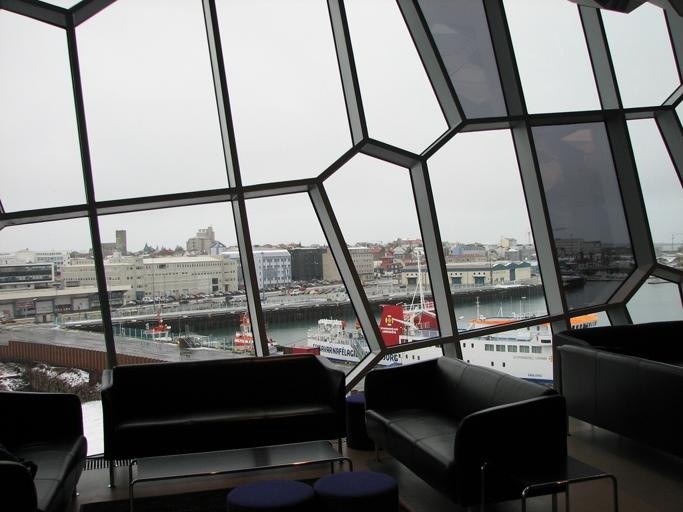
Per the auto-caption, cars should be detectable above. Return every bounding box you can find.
[126,286,305,307]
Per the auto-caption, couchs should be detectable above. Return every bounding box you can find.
[0,390,86,512]
[553,320,683,391]
[364,356,568,512]
[556,345,683,458]
[100,354,347,488]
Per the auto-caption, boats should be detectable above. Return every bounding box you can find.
[145,310,321,356]
[306,250,600,385]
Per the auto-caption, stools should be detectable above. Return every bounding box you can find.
[314,471,399,512]
[227,480,314,512]
[346,393,385,452]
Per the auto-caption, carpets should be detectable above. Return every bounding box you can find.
[80,477,413,512]
